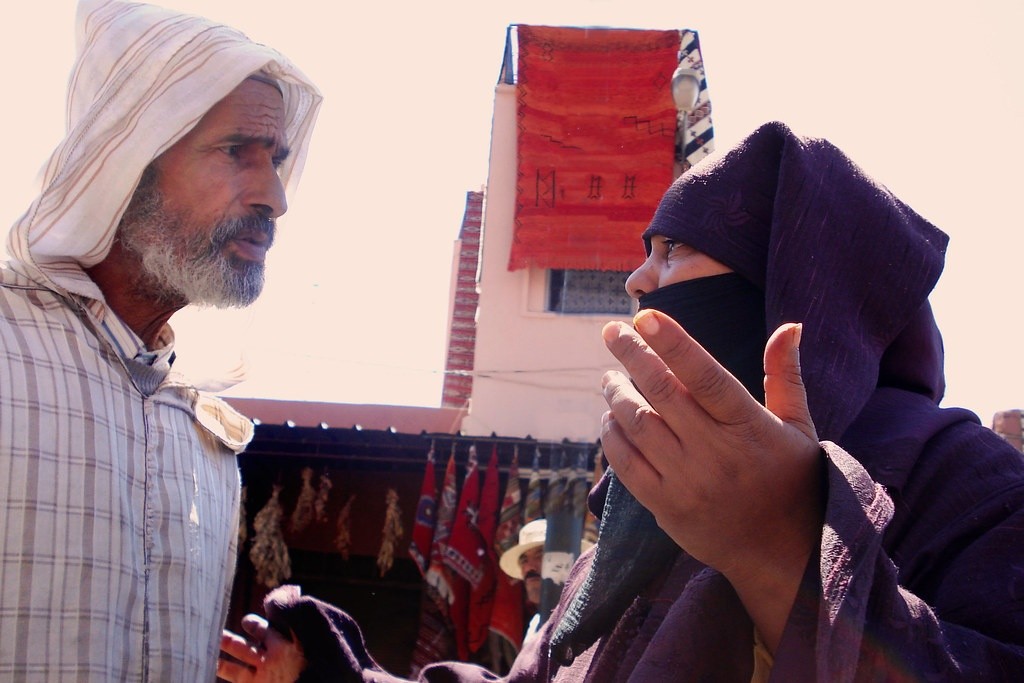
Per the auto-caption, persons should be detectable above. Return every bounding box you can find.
[217,117,1024,683]
[496,518,560,679]
[989,409,1024,455]
[0,2,324,683]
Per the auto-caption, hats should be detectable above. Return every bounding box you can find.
[499,519,595,580]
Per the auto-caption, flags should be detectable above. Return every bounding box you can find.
[407,436,608,660]
